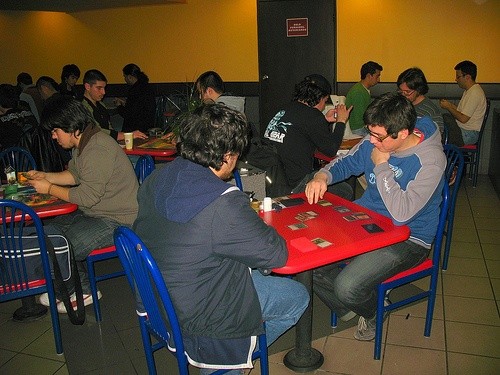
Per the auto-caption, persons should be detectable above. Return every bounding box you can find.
[25,99,141,313]
[440,61,487,187]
[345,61,383,137]
[132,100,311,358]
[298,90,447,341]
[263,74,356,202]
[0,64,158,173]
[396,68,445,134]
[194,71,246,113]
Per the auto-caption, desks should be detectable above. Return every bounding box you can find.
[256,192,410,373]
[120,136,176,157]
[0,183,78,322]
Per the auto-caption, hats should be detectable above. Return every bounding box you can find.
[307,74,333,105]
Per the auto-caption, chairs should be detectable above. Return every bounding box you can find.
[0,96,271,375]
[243,136,292,197]
[331,99,491,360]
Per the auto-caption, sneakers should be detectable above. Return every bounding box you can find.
[56,290,102,313]
[449,166,457,185]
[354,299,392,340]
[39,292,60,306]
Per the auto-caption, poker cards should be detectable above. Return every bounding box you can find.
[311,238,331,248]
[361,223,384,234]
[317,200,332,207]
[332,206,370,222]
[294,210,318,222]
[287,223,308,231]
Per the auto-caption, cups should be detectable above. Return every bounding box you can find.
[123,132,134,149]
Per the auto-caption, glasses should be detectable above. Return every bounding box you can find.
[363,125,399,142]
[456,72,468,79]
[396,88,416,96]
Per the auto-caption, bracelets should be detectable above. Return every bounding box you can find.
[48,184,55,195]
